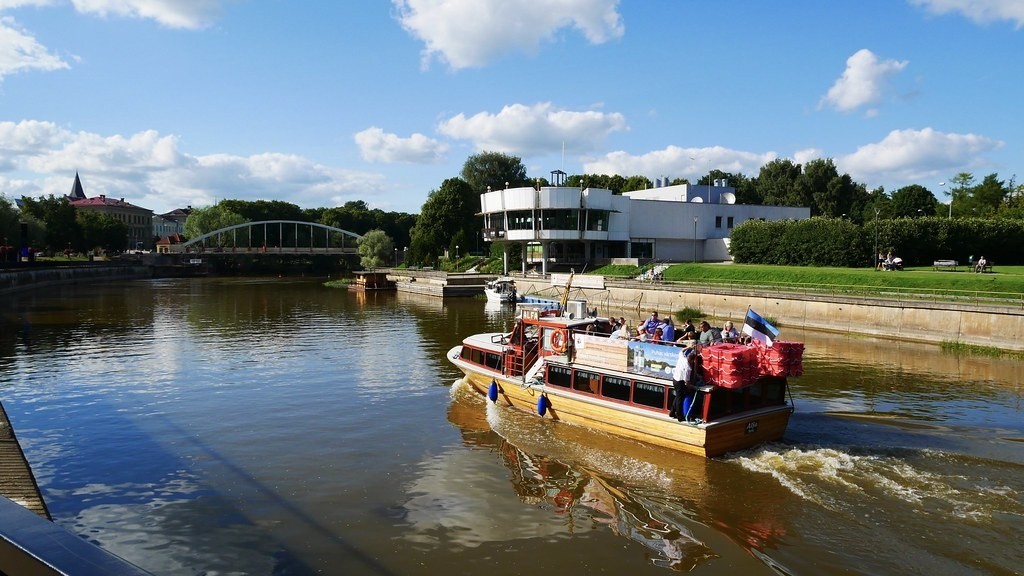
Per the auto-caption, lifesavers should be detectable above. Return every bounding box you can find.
[550,329,569,354]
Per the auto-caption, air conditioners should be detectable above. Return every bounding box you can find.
[567,301,587,318]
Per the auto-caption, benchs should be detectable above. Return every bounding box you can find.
[932,260,958,272]
[968,261,994,272]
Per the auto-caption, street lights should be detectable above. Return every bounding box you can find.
[938,182,953,220]
[693,215,698,263]
[455,245,460,271]
[476,230,479,256]
[689,156,711,202]
[395,248,399,267]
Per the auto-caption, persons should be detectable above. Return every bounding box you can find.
[669,342,695,422]
[974,256,987,273]
[584,310,739,347]
[877,250,902,271]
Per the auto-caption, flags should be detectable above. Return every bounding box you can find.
[742,306,780,349]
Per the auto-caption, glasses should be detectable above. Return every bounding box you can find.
[652,315,656,316]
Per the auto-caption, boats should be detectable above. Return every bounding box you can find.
[447,272,807,459]
[484,276,517,303]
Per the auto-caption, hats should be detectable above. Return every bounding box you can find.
[726,321,733,326]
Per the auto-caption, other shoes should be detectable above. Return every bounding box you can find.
[669,413,677,418]
[678,418,686,421]
